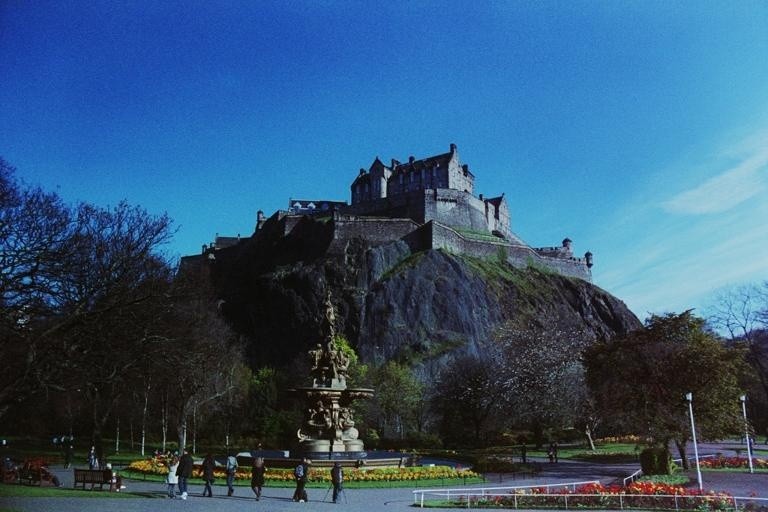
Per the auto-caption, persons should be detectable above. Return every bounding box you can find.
[103,463,126,492]
[331,461,343,503]
[89,446,95,467]
[521,443,526,463]
[226,453,238,496]
[292,457,311,503]
[60,434,74,469]
[198,453,215,497]
[166,448,193,500]
[308,400,355,430]
[548,442,558,463]
[251,457,265,500]
[308,291,350,381]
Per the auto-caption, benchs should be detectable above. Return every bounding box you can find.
[19,468,49,488]
[73,468,117,493]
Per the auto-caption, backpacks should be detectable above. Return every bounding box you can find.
[296,465,304,477]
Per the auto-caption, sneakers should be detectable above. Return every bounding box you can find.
[167,489,341,504]
[116,486,126,491]
